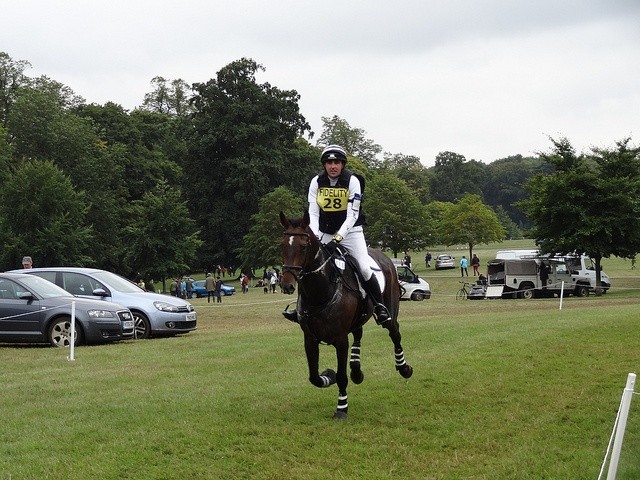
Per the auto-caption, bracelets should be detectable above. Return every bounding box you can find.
[332,234,342,244]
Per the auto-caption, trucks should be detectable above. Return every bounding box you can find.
[486,260,575,299]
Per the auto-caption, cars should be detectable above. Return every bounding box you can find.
[191,280,235,298]
[0,273,135,347]
[4,268,197,340]
[434,255,455,269]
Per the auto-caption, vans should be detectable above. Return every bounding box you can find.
[495,250,610,294]
[394,264,431,301]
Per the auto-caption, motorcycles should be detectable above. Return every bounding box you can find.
[469,281,487,299]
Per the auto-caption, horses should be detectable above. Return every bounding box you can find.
[278,212,413,420]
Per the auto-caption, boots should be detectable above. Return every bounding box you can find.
[362,272,388,324]
[282,308,299,321]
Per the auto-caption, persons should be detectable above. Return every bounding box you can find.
[22,256,33,269]
[169,277,194,298]
[254,269,268,288]
[204,272,216,304]
[69,278,84,295]
[268,266,279,294]
[424,250,432,268]
[138,278,145,289]
[426,250,428,255]
[479,274,486,284]
[471,254,480,277]
[538,261,550,293]
[403,250,412,269]
[203,264,235,277]
[282,145,389,322]
[214,276,224,304]
[240,272,249,294]
[460,256,468,277]
[148,279,156,293]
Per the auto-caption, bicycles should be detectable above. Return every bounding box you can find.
[456,281,475,302]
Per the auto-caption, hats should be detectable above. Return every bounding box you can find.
[22,256,32,264]
[320,145,348,162]
